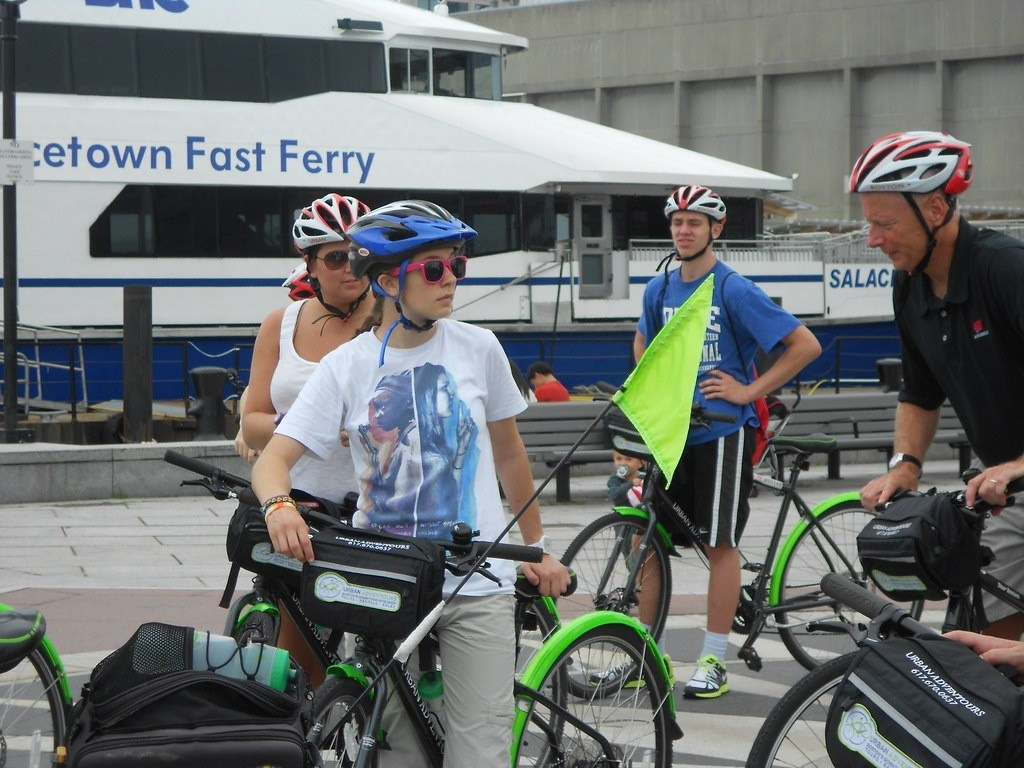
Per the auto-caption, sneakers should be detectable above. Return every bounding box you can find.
[589,654,676,688]
[684,656,729,697]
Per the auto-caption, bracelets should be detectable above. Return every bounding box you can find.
[261,495,297,522]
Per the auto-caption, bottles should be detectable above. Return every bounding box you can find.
[132,623,297,693]
[417,671,448,741]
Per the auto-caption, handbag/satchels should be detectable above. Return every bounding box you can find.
[54,623,326,768]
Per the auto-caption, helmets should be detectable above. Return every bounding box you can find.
[665,185,727,225]
[282,262,315,300]
[849,131,974,194]
[292,193,371,253]
[345,200,478,279]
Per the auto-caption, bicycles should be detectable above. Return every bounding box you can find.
[745,468,1024,768]
[0,600,74,768]
[165,450,684,768]
[543,382,925,701]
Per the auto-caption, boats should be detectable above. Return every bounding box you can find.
[0,0,908,403]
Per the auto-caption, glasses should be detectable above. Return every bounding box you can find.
[312,251,348,270]
[385,256,467,282]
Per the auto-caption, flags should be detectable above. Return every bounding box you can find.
[615,274,714,489]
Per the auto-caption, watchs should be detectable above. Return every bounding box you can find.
[273,412,287,426]
[529,536,552,555]
[889,452,923,480]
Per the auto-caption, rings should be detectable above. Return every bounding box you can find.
[990,479,996,483]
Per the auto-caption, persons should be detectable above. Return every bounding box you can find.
[252,201,573,768]
[527,362,569,402]
[234,194,377,692]
[607,450,644,617]
[510,361,537,403]
[588,185,822,697]
[851,131,1024,622]
[943,630,1024,672]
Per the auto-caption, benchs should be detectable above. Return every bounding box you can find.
[512,391,974,501]
